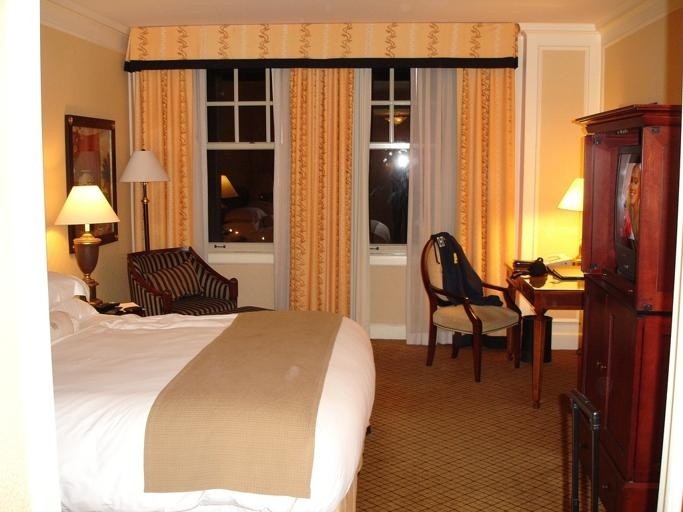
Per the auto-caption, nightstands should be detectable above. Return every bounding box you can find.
[105,307,147,317]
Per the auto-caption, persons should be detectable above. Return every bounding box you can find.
[392,164,407,243]
[624,163,642,241]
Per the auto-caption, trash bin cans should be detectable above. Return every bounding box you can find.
[523,314,552,362]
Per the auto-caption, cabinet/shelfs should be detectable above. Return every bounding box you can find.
[576,104,682,512]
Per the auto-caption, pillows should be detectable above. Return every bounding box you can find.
[142,259,202,301]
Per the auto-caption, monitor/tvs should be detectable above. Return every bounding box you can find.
[614,144,642,281]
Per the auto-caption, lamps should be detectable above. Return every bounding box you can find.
[119,148,172,251]
[54,186,120,313]
[556,178,584,265]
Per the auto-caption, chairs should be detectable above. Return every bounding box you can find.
[421,239,521,382]
[126,246,238,315]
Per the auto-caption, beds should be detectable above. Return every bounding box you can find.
[49,271,376,512]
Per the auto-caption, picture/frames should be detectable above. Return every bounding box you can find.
[65,114,118,253]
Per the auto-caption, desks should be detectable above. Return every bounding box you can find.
[505,262,585,409]
